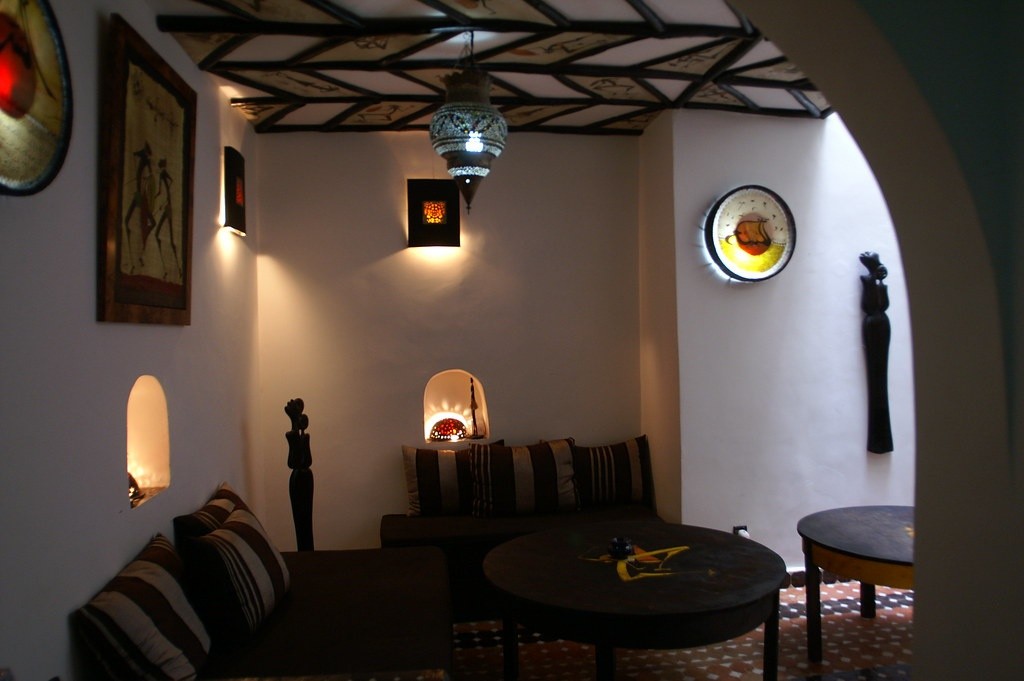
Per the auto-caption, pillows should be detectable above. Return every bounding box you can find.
[72,533,212,681]
[469,436,582,519]
[572,433,654,512]
[172,480,295,636]
[401,438,506,516]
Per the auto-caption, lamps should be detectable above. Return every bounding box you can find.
[0,0,75,192]
[429,29,509,214]
[699,185,797,284]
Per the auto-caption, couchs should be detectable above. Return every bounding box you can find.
[198,550,454,681]
[381,505,667,565]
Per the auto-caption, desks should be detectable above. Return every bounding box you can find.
[797,505,916,661]
[481,519,789,681]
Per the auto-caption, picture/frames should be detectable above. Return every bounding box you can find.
[95,14,198,324]
